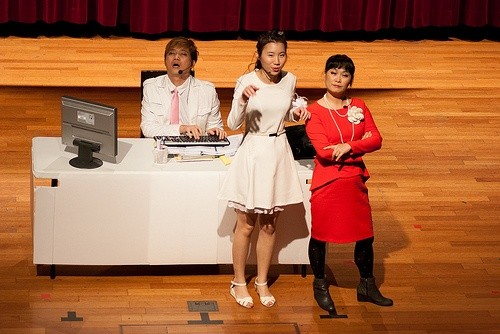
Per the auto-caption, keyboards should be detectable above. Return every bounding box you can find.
[153,133,230,147]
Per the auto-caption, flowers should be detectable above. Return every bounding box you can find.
[347,105,364,125]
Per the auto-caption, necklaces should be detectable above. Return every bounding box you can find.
[322,94,355,144]
[263,69,272,80]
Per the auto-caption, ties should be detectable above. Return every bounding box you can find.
[170,89,180,126]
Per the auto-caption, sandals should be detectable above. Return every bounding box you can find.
[230,280,253,308]
[253,277,276,308]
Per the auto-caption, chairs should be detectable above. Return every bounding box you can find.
[140,70,195,138]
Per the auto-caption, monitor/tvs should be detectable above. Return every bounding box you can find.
[61,95,118,169]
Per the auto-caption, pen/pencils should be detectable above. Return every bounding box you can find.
[200,153,224,156]
[154,137,166,150]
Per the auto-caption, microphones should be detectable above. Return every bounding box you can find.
[178,63,193,74]
[342,95,349,100]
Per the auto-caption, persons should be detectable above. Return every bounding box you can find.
[225,32,311,308]
[305,55,393,313]
[140,38,227,141]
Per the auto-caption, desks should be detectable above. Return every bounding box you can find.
[32,134,316,278]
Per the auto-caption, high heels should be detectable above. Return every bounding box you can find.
[356,277,394,307]
[312,276,335,311]
